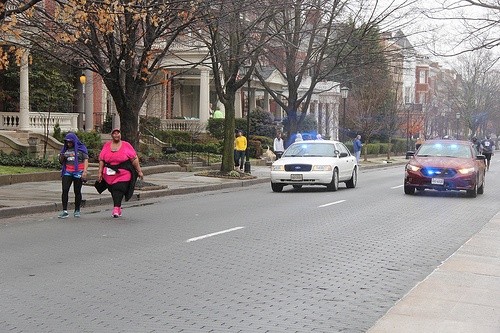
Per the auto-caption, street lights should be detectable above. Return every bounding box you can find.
[341,86,349,144]
[406,103,411,159]
[455,113,460,140]
[244,65,253,174]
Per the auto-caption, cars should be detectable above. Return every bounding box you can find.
[404,140,486,198]
[271,140,358,192]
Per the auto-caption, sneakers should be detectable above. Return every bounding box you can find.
[74,210,80,217]
[58,210,69,218]
[111,206,122,217]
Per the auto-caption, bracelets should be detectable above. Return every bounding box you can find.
[84,171,87,173]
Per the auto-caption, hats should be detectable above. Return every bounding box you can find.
[111,128,120,134]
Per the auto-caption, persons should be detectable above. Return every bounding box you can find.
[317,134,324,140]
[470,136,480,155]
[481,135,495,171]
[415,135,425,150]
[273,133,284,161]
[295,133,303,143]
[209,103,214,118]
[235,131,247,171]
[214,107,223,118]
[234,134,237,166]
[95,128,143,218]
[58,133,89,219]
[352,135,363,166]
